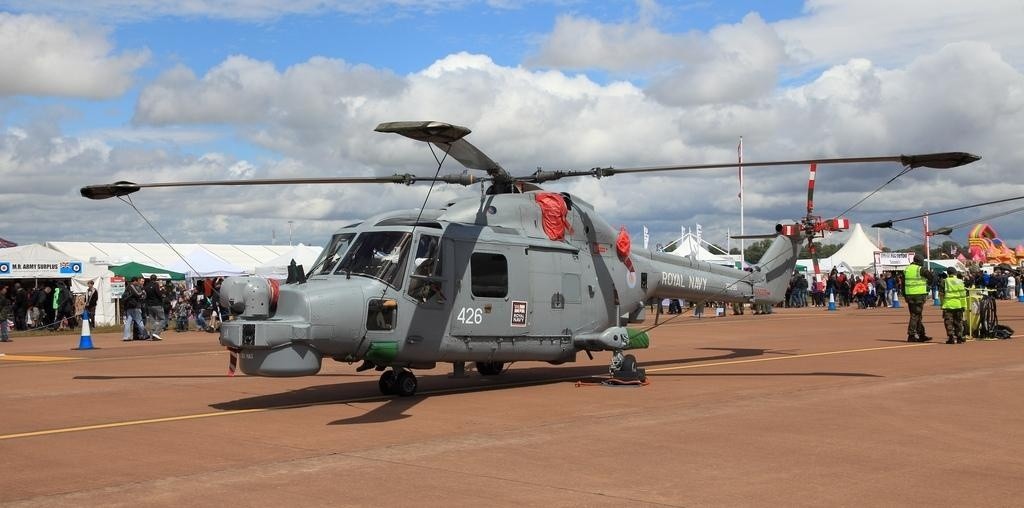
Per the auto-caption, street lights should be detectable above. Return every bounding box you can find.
[285,217,295,244]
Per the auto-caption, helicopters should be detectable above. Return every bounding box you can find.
[81,120,982,395]
[871,195,1024,236]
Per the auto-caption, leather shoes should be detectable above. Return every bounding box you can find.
[957,338,964,344]
[908,335,919,343]
[919,335,932,341]
[152,334,163,341]
[946,338,954,344]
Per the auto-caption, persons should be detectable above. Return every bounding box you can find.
[777,271,809,308]
[668,298,773,315]
[812,268,905,309]
[901,254,933,342]
[938,267,968,343]
[370,243,420,326]
[929,269,1024,301]
[0,274,225,343]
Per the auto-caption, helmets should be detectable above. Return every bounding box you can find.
[915,254,925,264]
[947,266,958,275]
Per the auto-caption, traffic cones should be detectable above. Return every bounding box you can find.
[932,286,941,306]
[71,311,101,350]
[826,287,838,310]
[1017,282,1024,301]
[889,288,904,308]
